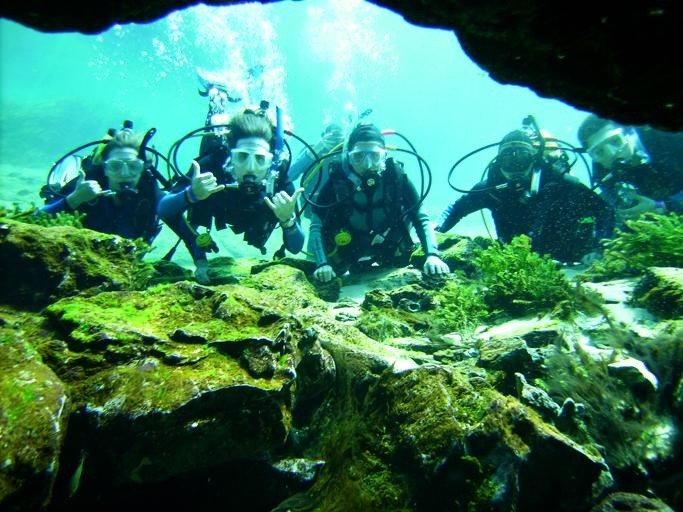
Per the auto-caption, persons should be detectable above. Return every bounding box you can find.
[576,113,683,226]
[308,122,450,282]
[155,69,304,255]
[409,129,612,268]
[32,128,212,285]
[286,120,343,215]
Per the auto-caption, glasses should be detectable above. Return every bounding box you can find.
[499,149,535,170]
[348,149,385,165]
[105,158,144,177]
[587,128,628,162]
[230,148,273,170]
